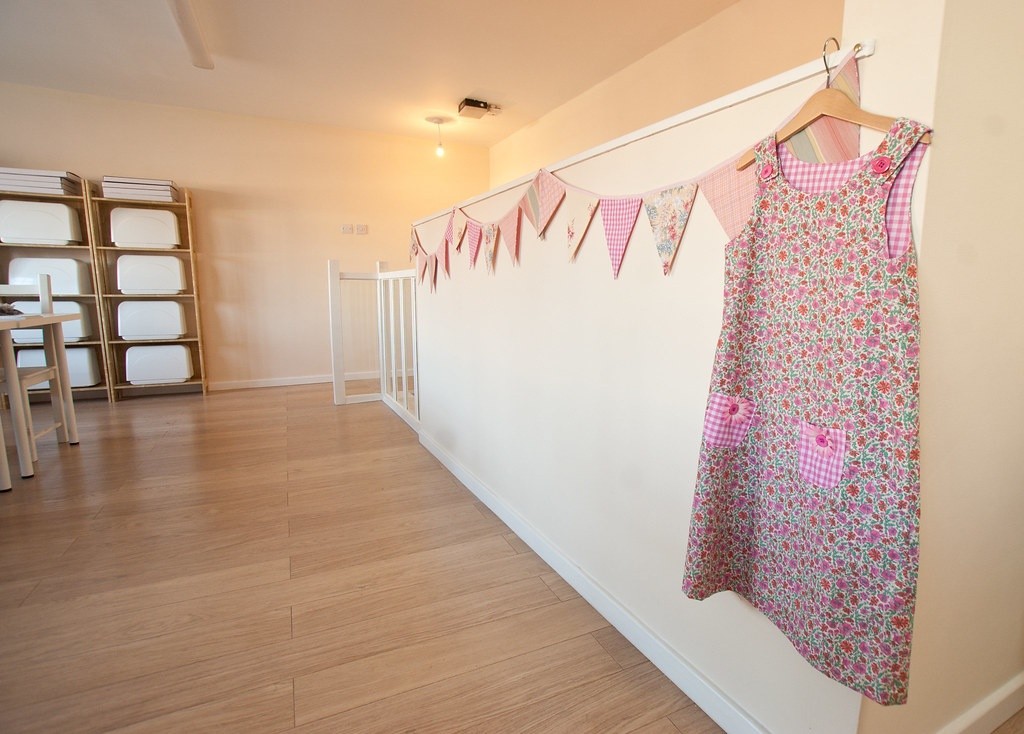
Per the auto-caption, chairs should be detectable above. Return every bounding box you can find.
[0,274,68,462]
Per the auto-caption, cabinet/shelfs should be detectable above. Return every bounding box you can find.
[0,180,209,403]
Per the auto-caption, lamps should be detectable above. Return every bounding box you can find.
[169,0,214,69]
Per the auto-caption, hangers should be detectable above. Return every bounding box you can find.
[738,36,933,171]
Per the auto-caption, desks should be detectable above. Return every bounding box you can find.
[0,314,83,493]
